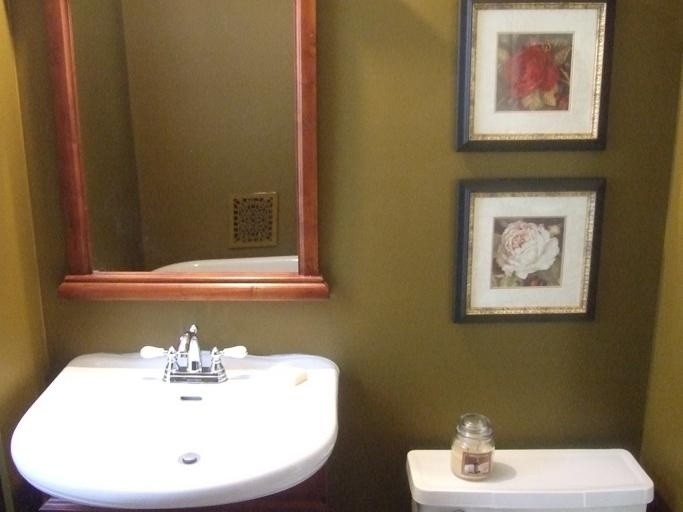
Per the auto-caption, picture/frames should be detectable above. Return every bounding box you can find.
[452,176,607,322]
[457,0,615,153]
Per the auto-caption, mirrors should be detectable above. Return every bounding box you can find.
[37,0,331,302]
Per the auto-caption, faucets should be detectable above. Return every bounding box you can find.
[188,326,201,376]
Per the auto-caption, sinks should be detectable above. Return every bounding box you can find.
[154,257,298,274]
[9,353,339,508]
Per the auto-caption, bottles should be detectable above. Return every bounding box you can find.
[450,411,496,481]
